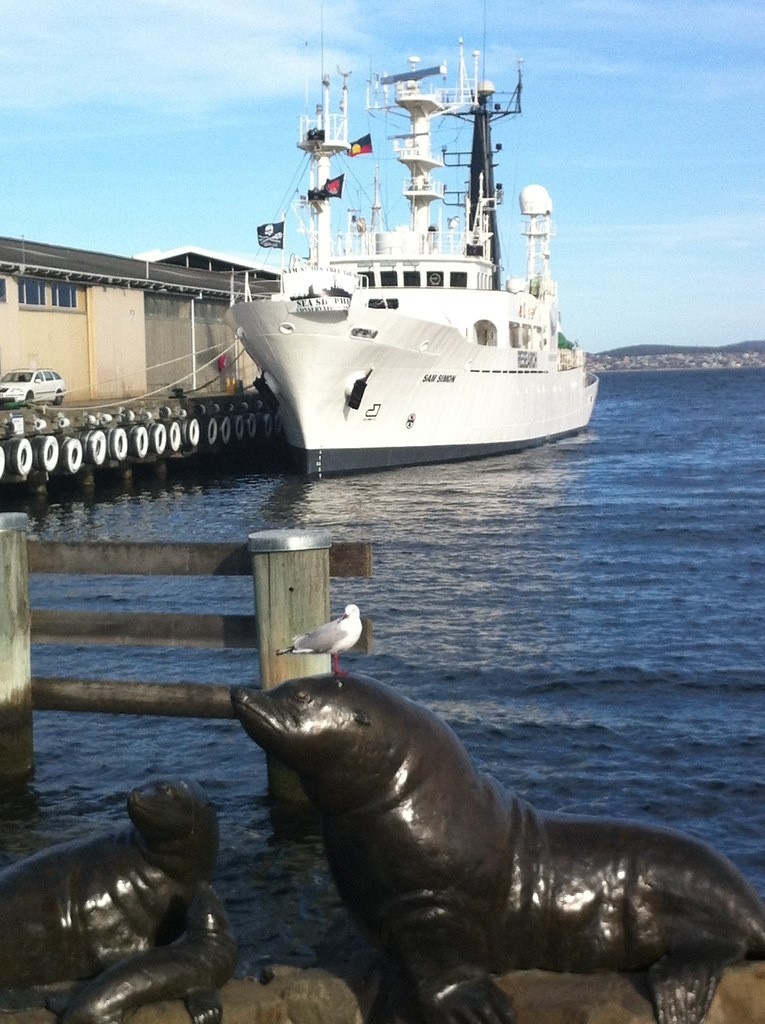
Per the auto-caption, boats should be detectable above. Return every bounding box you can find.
[224,38,599,475]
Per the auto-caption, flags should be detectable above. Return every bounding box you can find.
[324,173,345,199]
[257,221,284,249]
[346,132,373,157]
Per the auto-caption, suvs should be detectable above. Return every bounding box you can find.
[0,368,66,409]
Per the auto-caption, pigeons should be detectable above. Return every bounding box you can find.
[273,604,362,678]
